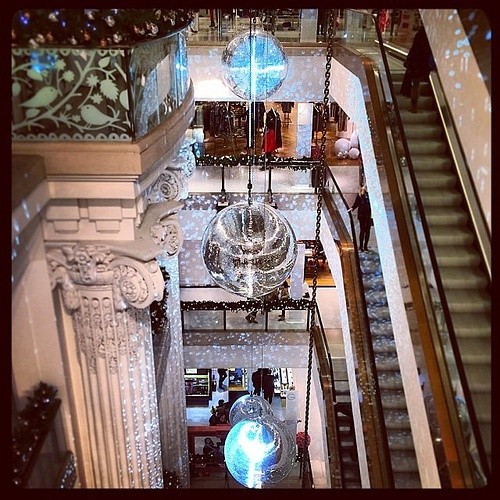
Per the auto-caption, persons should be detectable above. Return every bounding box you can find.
[277,287,288,321]
[260,108,282,153]
[347,186,374,252]
[399,19,436,114]
[203,438,217,454]
[332,401,358,461]
[252,368,262,396]
[245,308,258,324]
[262,370,275,404]
[218,368,228,390]
